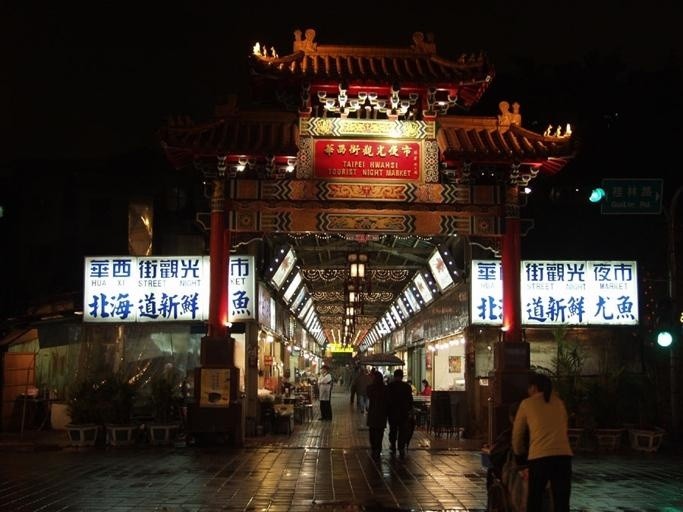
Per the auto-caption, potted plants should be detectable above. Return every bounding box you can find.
[66,373,113,448]
[101,375,139,455]
[149,378,182,446]
[630,373,667,452]
[593,359,630,462]
[528,324,588,456]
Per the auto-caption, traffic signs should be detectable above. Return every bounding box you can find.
[600,178,666,216]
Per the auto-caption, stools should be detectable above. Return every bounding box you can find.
[261,384,313,435]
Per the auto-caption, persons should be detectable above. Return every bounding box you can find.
[511,372,575,511]
[315,365,333,421]
[332,363,431,467]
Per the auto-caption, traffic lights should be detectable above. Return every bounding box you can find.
[545,177,607,210]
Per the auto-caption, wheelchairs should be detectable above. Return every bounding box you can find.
[480,445,529,511]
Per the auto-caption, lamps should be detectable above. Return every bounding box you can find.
[341,246,368,349]
[586,187,607,207]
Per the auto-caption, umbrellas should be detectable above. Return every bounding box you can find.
[357,352,406,366]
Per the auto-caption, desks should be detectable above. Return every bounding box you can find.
[16,398,61,433]
